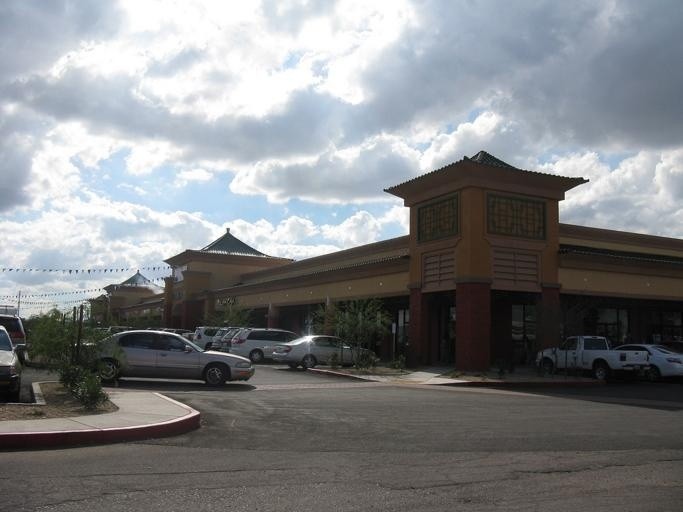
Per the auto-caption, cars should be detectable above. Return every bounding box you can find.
[0,325,26,402]
[611,344,683,383]
[271,334,380,369]
[70,330,255,388]
[219,327,302,364]
[83,326,231,353]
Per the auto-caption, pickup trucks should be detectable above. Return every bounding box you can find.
[535,335,650,381]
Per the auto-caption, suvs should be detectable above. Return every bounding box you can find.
[0,305,31,365]
